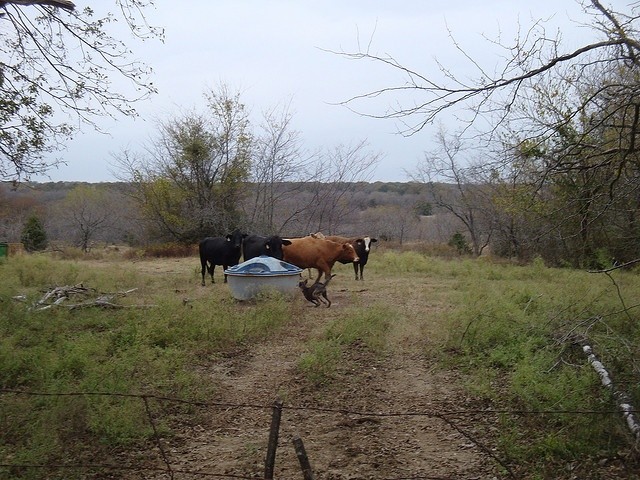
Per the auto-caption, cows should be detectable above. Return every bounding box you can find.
[198,228,249,286]
[324,234,378,281]
[281,235,360,282]
[242,234,292,262]
[308,231,327,279]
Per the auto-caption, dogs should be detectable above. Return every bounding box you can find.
[298,273,337,308]
